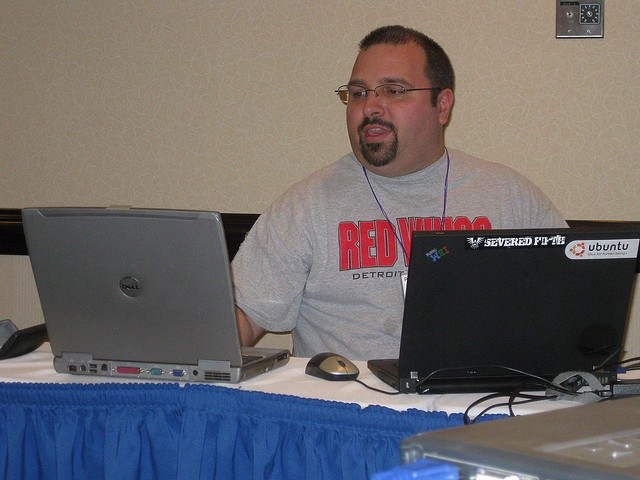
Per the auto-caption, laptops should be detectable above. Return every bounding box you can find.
[20,205,292,384]
[367,225,639,394]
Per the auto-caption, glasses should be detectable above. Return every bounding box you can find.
[334,84,434,104]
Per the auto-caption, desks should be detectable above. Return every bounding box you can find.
[0,348,640,479]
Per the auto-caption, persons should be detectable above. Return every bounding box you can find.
[229,25,570,361]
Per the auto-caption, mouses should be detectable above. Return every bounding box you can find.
[306,351,359,382]
